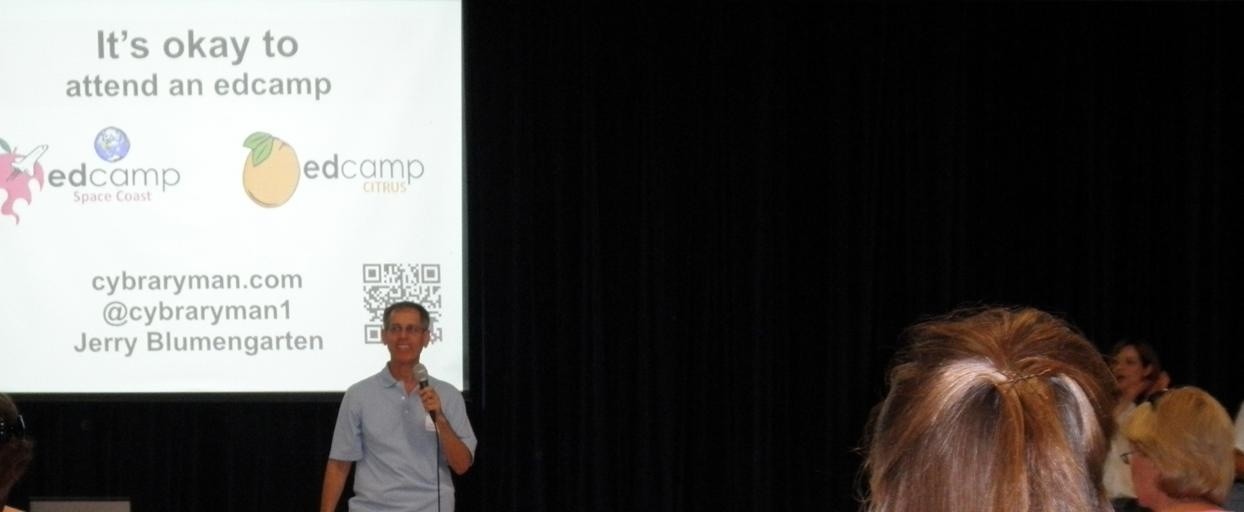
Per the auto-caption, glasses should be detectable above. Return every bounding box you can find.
[0,415,26,444]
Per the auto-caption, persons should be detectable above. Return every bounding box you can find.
[317,299,478,511]
[870,306,1122,511]
[1118,387,1236,512]
[1229,400,1243,473]
[1102,334,1172,512]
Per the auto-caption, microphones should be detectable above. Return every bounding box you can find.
[413,363,436,424]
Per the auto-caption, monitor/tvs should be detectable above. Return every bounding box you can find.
[30,501,131,512]
